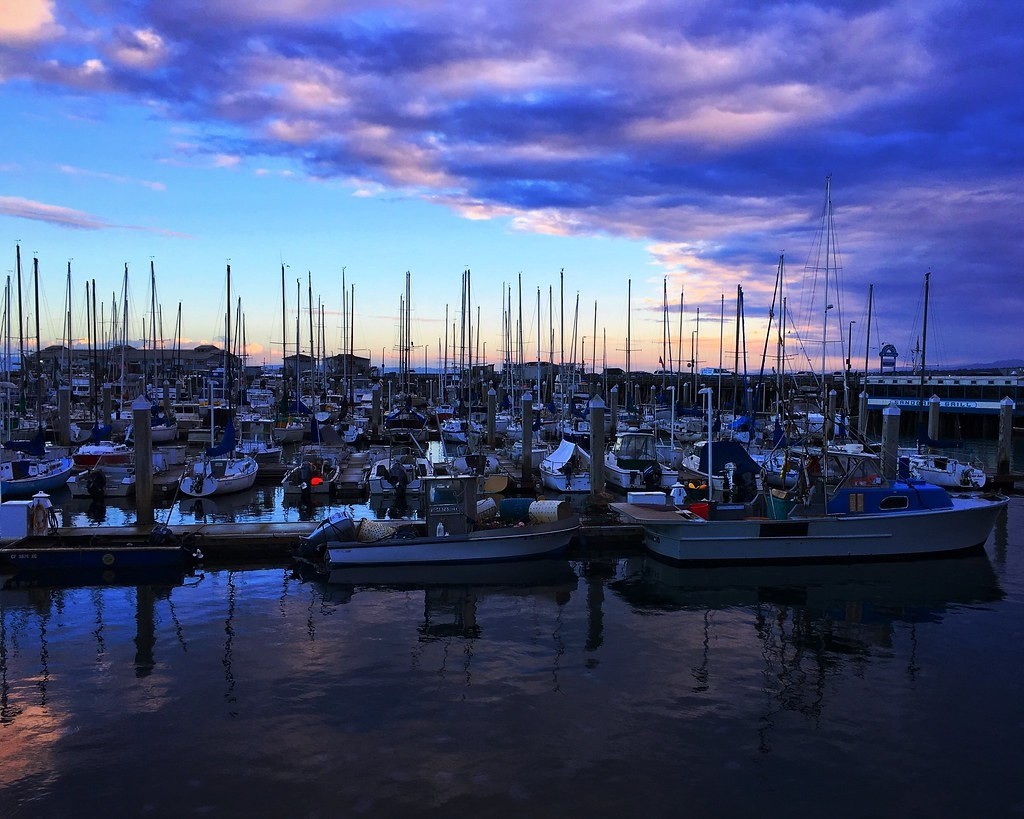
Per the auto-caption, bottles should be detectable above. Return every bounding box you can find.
[436,522,444,536]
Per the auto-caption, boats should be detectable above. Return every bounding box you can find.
[315,515,583,567]
[319,564,581,605]
[0,535,188,591]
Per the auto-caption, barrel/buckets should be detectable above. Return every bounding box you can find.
[689,503,708,521]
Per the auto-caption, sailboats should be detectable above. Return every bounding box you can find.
[0,241,988,510]
[606,173,1012,568]
[614,552,1009,729]
